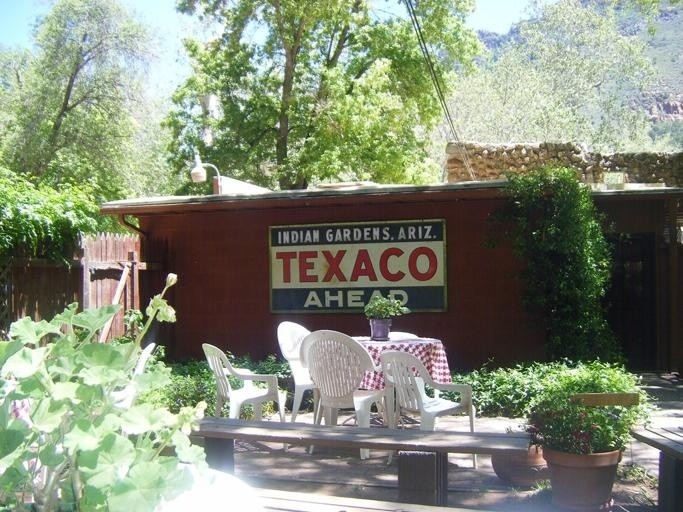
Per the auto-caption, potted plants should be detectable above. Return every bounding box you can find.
[362,295,412,340]
[471,363,550,487]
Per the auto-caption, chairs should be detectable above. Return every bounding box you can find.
[378,350,478,470]
[296,328,389,463]
[201,341,290,456]
[276,321,326,433]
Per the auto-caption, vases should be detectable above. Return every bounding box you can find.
[540,443,628,511]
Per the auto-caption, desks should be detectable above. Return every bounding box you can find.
[328,333,453,426]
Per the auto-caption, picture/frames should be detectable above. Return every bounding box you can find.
[267,217,449,314]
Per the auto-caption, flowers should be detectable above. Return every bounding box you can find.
[524,362,661,455]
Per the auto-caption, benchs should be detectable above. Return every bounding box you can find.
[190,419,535,506]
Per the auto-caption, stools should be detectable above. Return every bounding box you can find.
[631,423,683,510]
